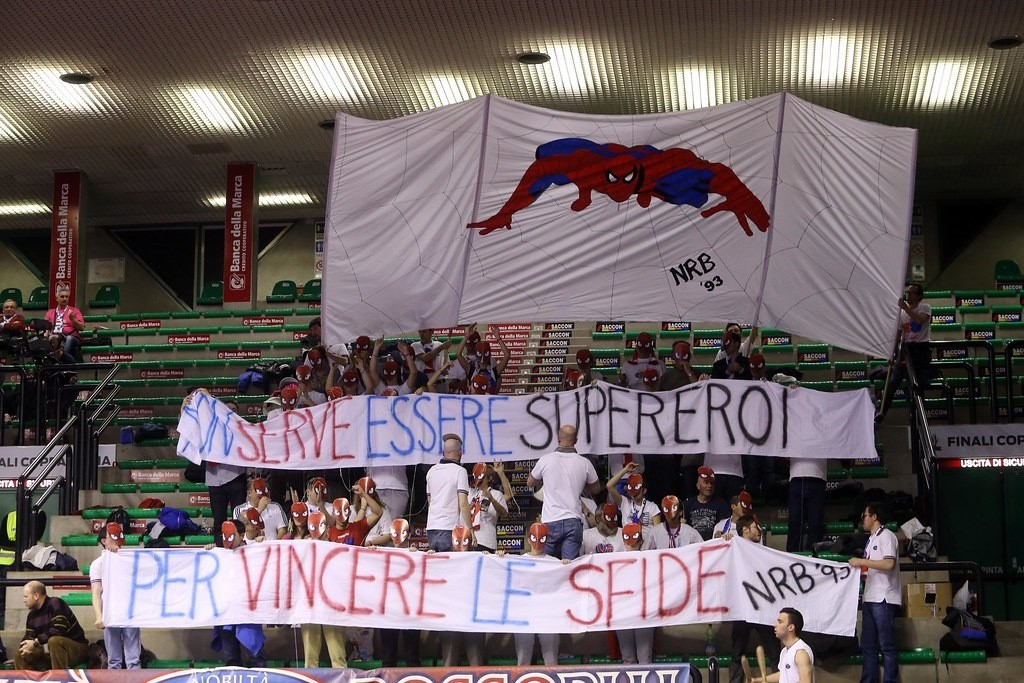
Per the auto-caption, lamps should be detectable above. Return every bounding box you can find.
[517,51,551,64]
[59,73,95,84]
[987,34,1024,51]
[318,119,335,131]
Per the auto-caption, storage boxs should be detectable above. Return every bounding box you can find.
[900,582,953,619]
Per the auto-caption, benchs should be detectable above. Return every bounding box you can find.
[0,289,1024,683]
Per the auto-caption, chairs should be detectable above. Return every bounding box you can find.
[299,279,321,302]
[0,287,22,311]
[266,279,297,302]
[86,284,120,307]
[993,259,1024,291]
[196,280,224,305]
[22,286,49,311]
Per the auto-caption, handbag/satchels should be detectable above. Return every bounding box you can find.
[184,461,206,484]
[134,422,169,442]
[0,509,46,548]
[120,426,136,444]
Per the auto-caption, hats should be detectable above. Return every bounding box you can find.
[442,434,462,445]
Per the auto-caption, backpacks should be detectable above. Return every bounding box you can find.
[138,498,165,508]
[908,529,938,563]
[158,507,199,531]
[939,605,996,652]
[868,366,894,380]
[246,361,296,382]
[106,506,130,534]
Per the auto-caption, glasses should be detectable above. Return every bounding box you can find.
[905,291,919,295]
[861,513,873,521]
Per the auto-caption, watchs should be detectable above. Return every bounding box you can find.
[33,639,40,648]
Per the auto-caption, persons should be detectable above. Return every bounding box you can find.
[0,284,958,683]
